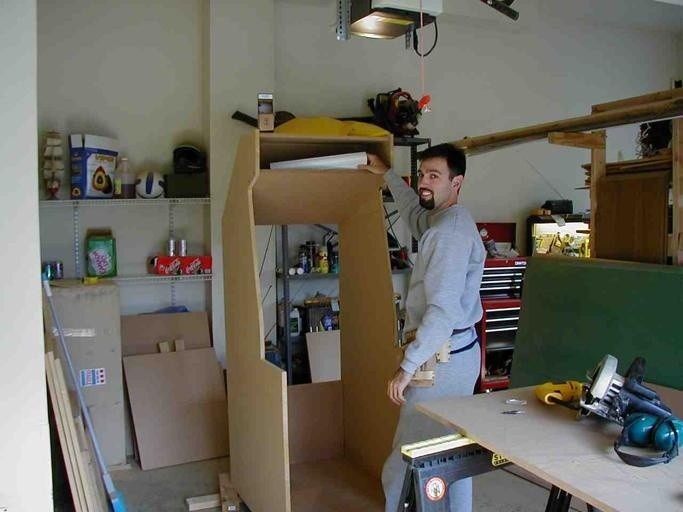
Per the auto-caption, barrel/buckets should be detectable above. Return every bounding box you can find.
[277,297,291,336]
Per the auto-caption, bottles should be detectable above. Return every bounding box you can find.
[479,227,497,256]
[305,239,317,268]
[297,244,308,274]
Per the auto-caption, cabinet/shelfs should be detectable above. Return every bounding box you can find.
[477,256,529,393]
[277,136,433,386]
[41,199,216,283]
[220,130,405,512]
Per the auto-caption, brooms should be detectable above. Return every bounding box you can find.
[41,271,127,512]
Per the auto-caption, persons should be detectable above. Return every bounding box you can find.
[357,142,486,512]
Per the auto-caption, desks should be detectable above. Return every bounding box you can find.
[414,385,683,512]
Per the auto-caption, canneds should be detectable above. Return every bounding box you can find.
[179,240,186,256]
[43,260,63,279]
[168,239,176,256]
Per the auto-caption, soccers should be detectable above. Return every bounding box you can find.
[135,170,165,198]
